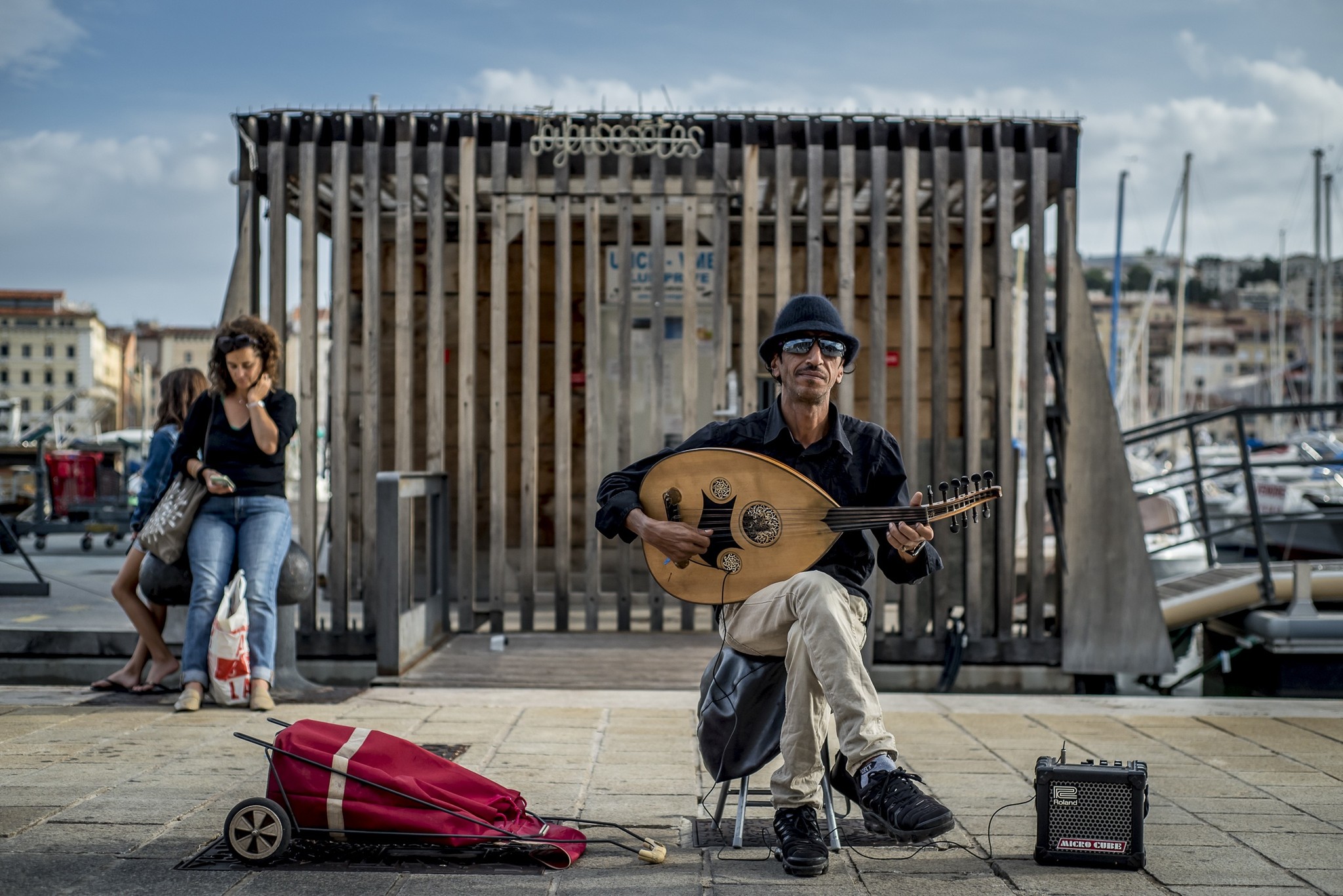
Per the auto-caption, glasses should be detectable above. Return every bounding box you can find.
[779,334,847,357]
[217,334,262,353]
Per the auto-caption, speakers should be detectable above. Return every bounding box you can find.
[1036,757,1148,870]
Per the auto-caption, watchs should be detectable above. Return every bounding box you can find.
[905,541,927,557]
[246,400,265,409]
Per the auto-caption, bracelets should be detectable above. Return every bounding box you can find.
[192,461,209,486]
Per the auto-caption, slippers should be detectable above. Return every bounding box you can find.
[90,678,129,692]
[129,681,182,696]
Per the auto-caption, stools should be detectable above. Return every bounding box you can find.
[694,648,843,854]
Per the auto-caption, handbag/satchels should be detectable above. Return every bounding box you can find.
[208,567,251,706]
[135,471,207,564]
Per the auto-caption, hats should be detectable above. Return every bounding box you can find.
[758,295,860,368]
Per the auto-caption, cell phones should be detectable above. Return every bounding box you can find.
[210,474,235,490]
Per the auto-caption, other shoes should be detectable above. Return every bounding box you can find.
[172,687,201,711]
[249,686,275,710]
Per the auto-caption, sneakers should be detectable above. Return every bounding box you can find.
[773,803,829,878]
[853,766,955,844]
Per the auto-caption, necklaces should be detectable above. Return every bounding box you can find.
[238,399,243,405]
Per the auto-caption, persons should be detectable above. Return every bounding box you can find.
[87,368,209,696]
[169,313,297,711]
[596,295,957,880]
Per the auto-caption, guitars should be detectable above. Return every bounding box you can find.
[636,446,1003,606]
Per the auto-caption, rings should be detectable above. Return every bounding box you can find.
[900,545,907,552]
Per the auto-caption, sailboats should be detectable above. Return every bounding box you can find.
[1011,145,1343,697]
